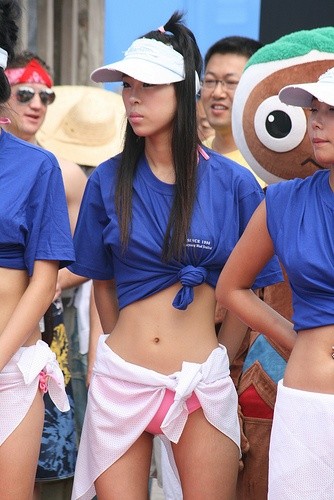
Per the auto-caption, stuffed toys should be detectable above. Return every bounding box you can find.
[221,24,333,500]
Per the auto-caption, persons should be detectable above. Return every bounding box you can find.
[55,278,105,450]
[67,11,282,499]
[215,63,334,500]
[191,90,217,150]
[188,35,275,499]
[0,48,89,499]
[1,1,90,500]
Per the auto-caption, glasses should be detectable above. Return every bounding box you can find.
[202,77,240,89]
[11,87,55,104]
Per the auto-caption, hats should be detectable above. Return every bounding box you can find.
[278,67,334,111]
[34,84,127,169]
[90,37,201,95]
